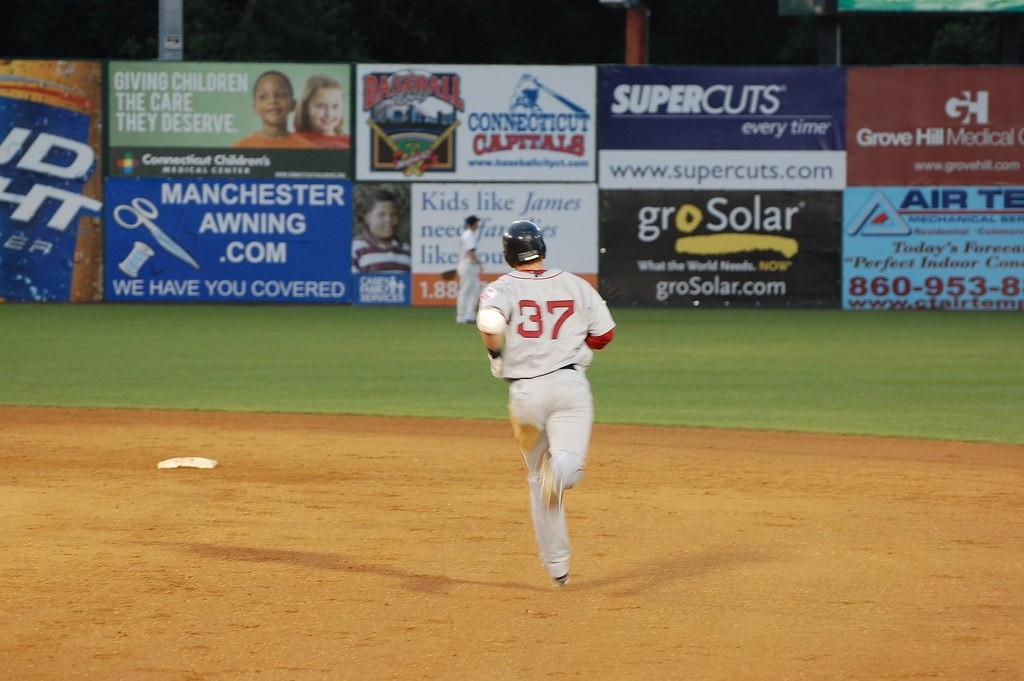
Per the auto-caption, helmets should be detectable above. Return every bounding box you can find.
[503,220,545,268]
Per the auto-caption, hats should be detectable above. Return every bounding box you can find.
[466,216,479,225]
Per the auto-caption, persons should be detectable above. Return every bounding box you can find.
[456,215,482,323]
[232,70,350,147]
[476,221,616,585]
[351,191,411,273]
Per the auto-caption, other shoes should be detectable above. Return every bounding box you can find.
[553,572,569,587]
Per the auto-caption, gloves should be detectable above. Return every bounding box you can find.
[490,352,506,380]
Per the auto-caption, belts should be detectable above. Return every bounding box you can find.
[510,364,574,381]
